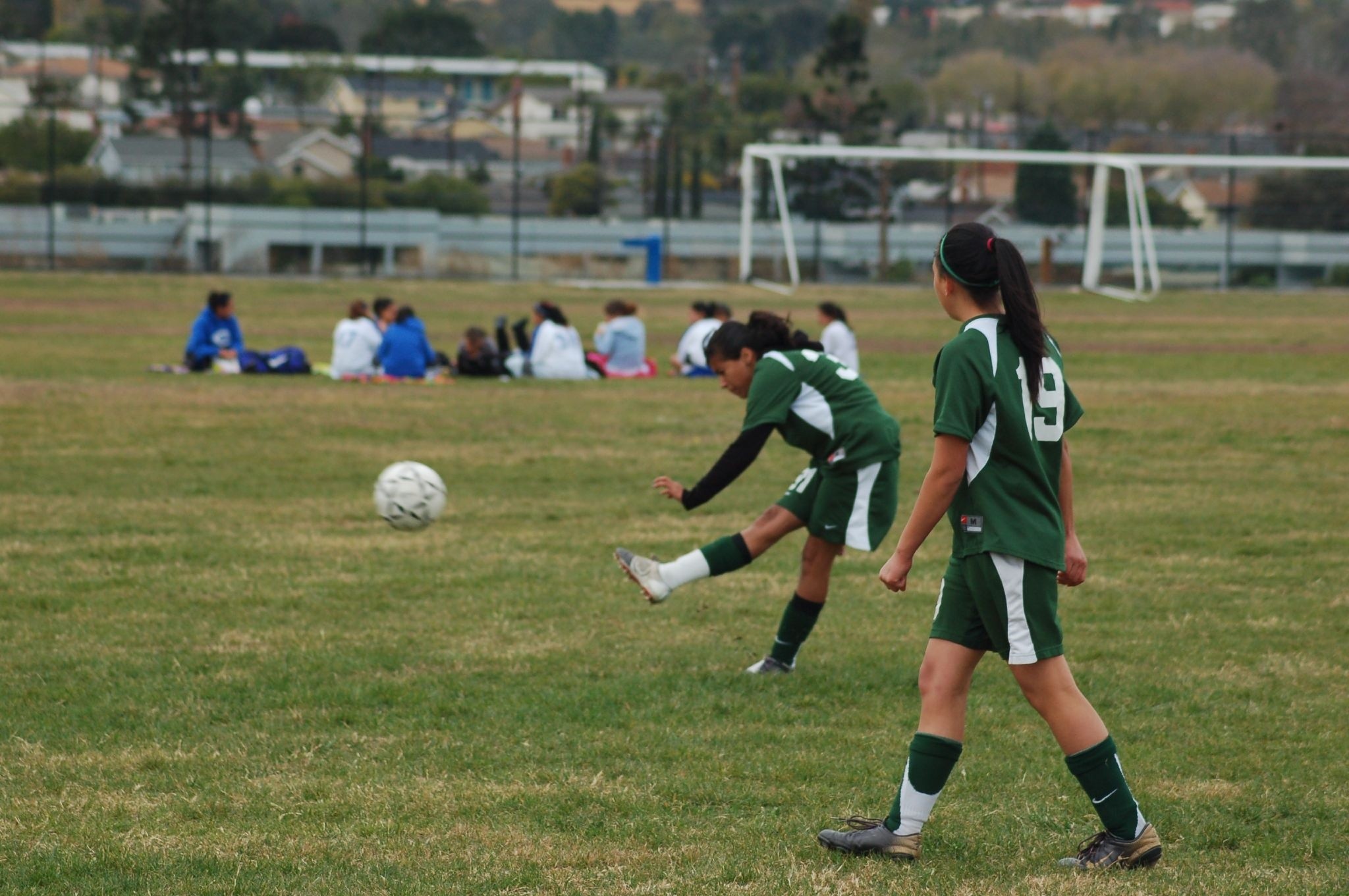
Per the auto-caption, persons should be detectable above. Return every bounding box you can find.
[183,292,245,371]
[334,297,447,384]
[815,222,1163,876]
[816,303,864,375]
[670,300,733,379]
[614,310,901,678]
[585,297,657,382]
[455,301,591,382]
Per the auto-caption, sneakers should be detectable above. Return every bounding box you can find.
[1056,823,1162,873]
[817,816,924,866]
[612,548,672,605]
[746,656,796,675]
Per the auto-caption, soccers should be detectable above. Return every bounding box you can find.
[373,460,446,531]
